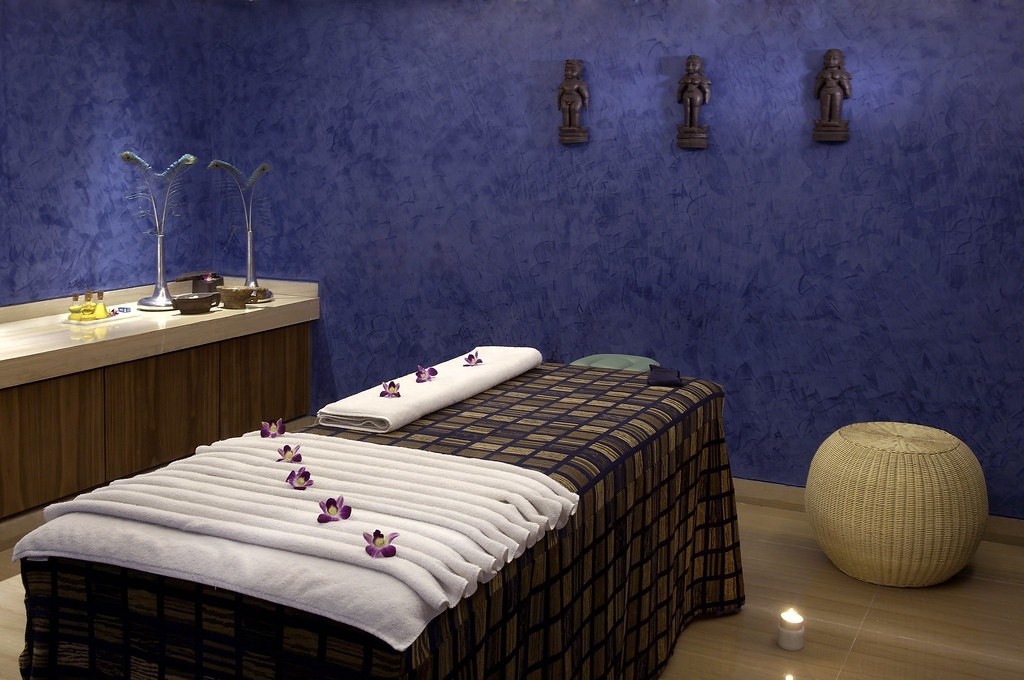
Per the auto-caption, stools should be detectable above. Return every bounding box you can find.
[569,354,661,372]
[804,422,987,588]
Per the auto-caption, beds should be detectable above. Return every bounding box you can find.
[18,359,745,680]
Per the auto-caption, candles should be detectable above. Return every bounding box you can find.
[777,608,805,651]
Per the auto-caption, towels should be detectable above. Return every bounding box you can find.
[11,424,581,653]
[317,344,544,433]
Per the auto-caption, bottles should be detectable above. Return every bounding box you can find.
[68,293,82,321]
[80,291,96,321]
[94,292,108,320]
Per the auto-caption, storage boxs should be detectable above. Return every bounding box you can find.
[192,275,224,302]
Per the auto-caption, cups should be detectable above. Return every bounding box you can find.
[216,286,266,309]
[192,275,224,301]
[172,292,221,315]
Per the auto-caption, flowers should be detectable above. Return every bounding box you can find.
[363,529,401,558]
[285,466,314,491]
[316,494,352,523]
[380,381,401,398]
[276,444,303,463]
[261,418,286,438]
[204,273,217,281]
[415,365,438,383]
[463,350,483,367]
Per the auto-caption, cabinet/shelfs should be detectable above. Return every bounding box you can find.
[0,275,320,552]
